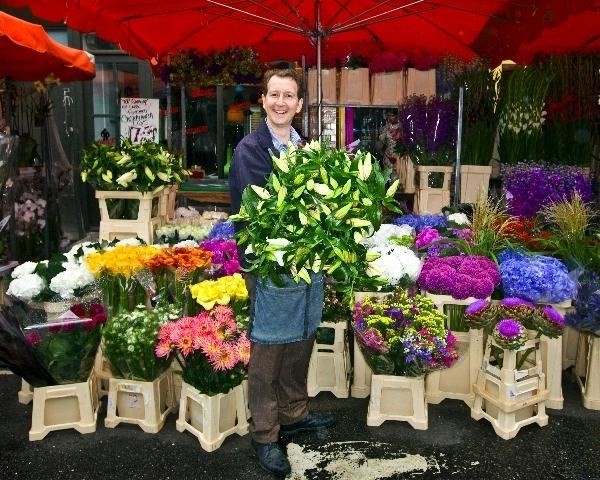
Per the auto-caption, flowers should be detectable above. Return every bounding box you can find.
[0,46,600,401]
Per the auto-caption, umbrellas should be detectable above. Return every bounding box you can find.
[0,10,99,87]
[466,0,599,72]
[6,1,499,160]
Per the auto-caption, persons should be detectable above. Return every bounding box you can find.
[226,67,339,475]
[379,108,400,178]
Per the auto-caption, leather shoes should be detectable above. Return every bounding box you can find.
[280,411,337,434]
[251,438,291,475]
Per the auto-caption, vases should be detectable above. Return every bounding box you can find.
[486,381,533,422]
[117,382,169,421]
[44,396,82,425]
[380,388,414,416]
[189,393,237,433]
